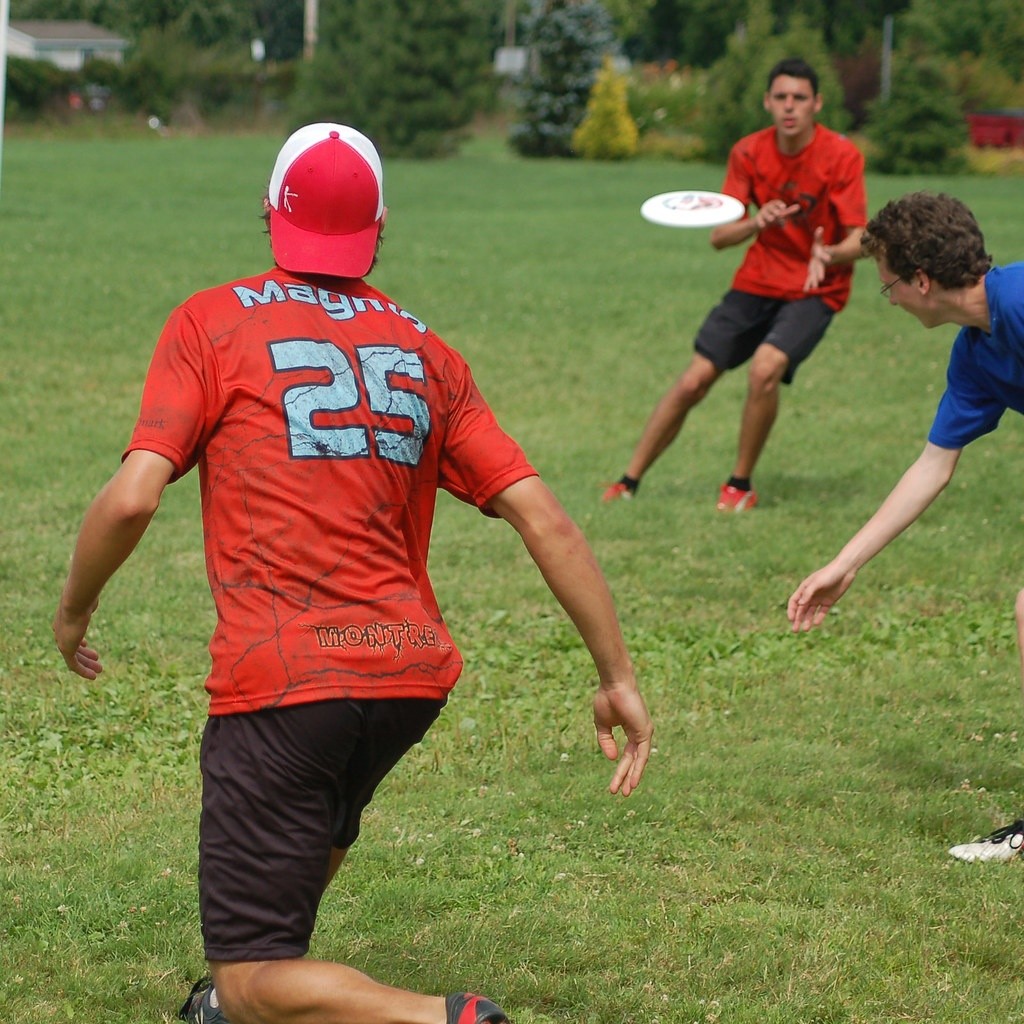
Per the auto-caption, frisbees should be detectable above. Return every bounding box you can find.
[639,190,746,229]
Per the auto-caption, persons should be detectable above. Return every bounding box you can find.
[599,55,867,513]
[54,125,654,1023]
[787,189,1023,864]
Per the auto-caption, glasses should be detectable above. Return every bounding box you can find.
[881,273,903,298]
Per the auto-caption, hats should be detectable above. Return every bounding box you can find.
[267,123,383,278]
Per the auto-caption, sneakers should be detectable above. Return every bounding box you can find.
[444,993,509,1024]
[716,485,757,509]
[178,976,229,1024]
[948,818,1024,863]
[605,482,629,501]
